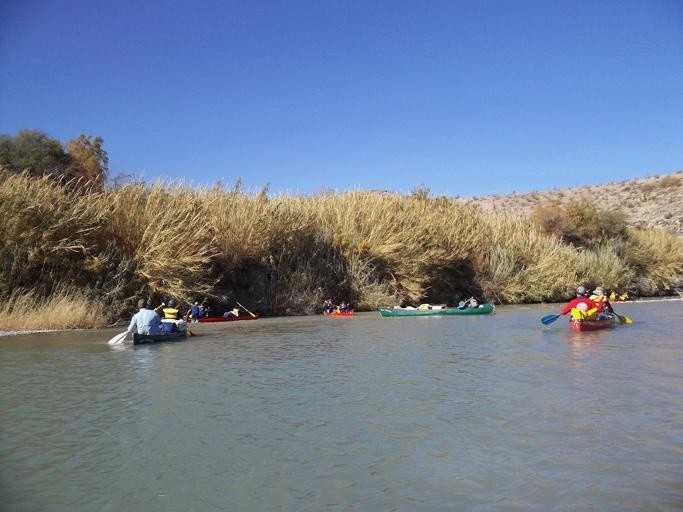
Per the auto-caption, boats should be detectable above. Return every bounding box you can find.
[132,314,259,345]
[324,302,495,317]
[567,317,615,332]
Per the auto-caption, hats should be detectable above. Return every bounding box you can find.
[592,286,604,296]
[137,299,146,308]
[168,300,176,307]
[576,286,585,294]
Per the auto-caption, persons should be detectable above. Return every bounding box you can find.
[128,298,208,335]
[561,286,630,320]
[324,299,349,313]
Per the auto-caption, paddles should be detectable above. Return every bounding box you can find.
[331,305,341,315]
[236,302,256,318]
[178,316,196,336]
[107,302,165,344]
[541,295,604,326]
[613,308,633,324]
